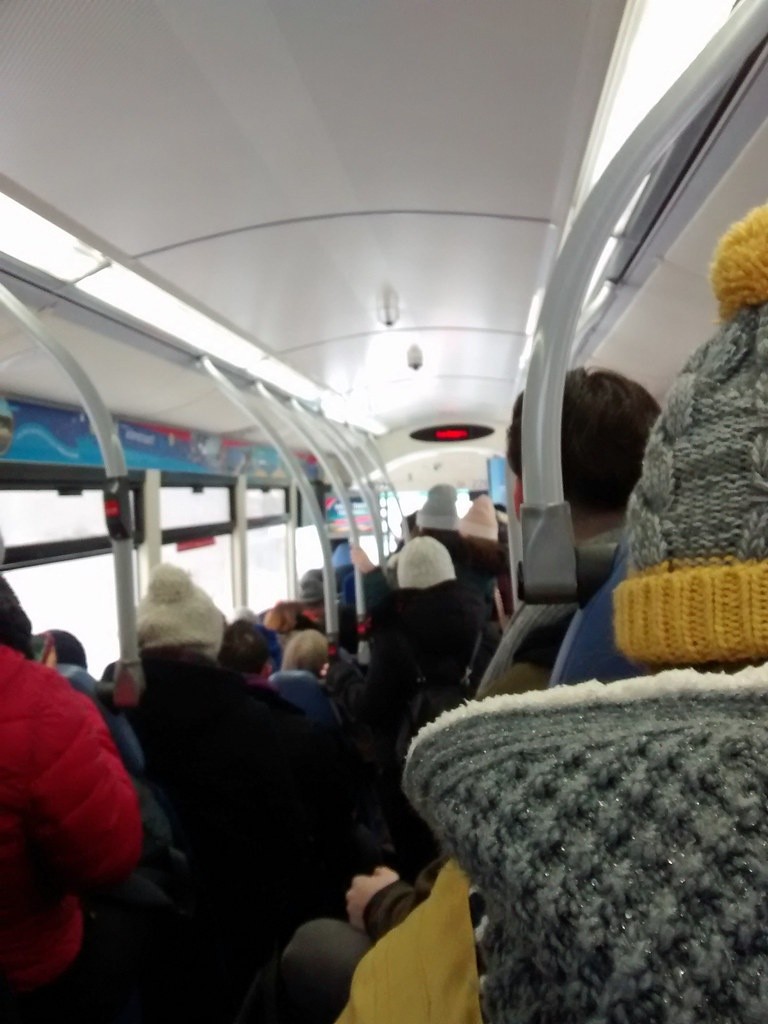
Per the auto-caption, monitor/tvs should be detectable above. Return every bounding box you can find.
[321,488,382,539]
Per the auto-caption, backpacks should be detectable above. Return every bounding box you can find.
[396,624,484,736]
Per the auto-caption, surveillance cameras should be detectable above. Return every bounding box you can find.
[407,347,423,370]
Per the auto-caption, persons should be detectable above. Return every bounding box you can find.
[1,367,664,1024]
[337,201,768,1024]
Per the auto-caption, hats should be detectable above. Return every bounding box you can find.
[44,629,88,671]
[300,569,323,603]
[612,201,768,666]
[459,493,499,541]
[415,484,461,532]
[331,542,352,568]
[396,535,457,590]
[134,562,225,652]
